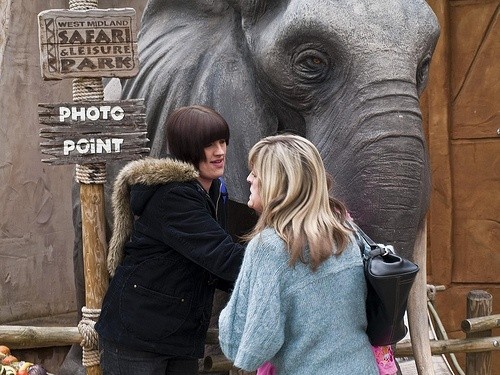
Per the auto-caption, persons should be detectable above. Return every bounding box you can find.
[92,105,353,374]
[225,133,409,374]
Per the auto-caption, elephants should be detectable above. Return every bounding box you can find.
[59,1,442,375]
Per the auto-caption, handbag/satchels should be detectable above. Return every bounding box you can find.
[351,222,420,346]
